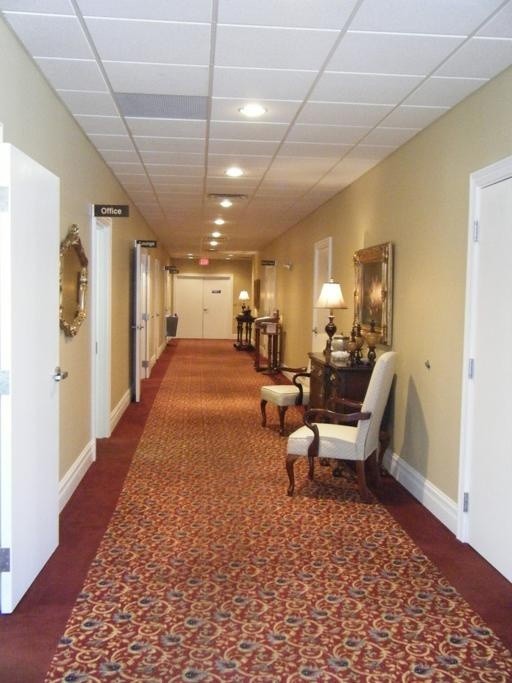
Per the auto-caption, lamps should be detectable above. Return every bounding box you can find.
[313,281,348,354]
[239,288,250,315]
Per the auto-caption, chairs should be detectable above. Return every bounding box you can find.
[258,330,345,436]
[282,349,403,500]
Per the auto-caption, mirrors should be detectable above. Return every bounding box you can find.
[58,218,97,337]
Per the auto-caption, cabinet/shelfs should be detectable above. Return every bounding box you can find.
[303,349,390,481]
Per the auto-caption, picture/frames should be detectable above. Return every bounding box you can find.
[352,240,396,347]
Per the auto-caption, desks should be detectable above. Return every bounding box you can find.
[253,316,285,374]
[234,313,255,351]
